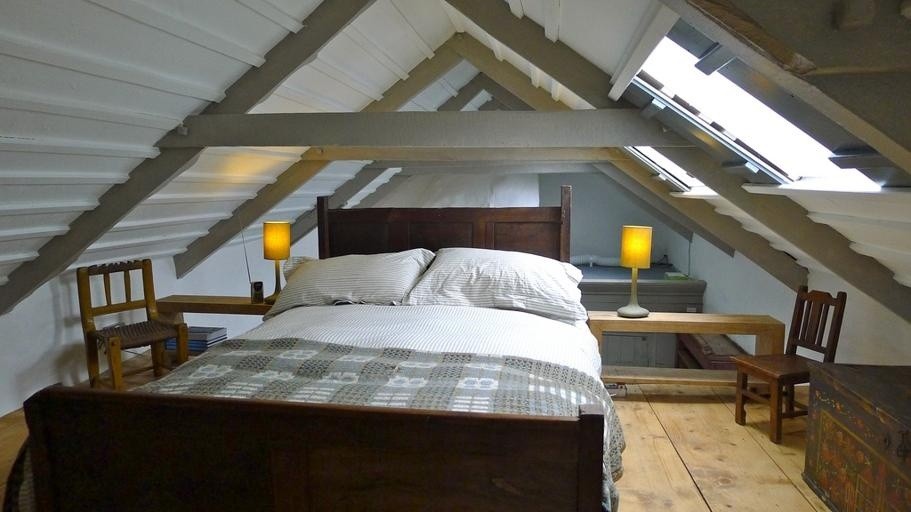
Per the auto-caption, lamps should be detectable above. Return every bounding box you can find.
[616,225,653,319]
[263,221,291,305]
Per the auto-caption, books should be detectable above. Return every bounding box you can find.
[166,326,228,352]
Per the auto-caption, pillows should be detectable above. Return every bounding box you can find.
[263,248,436,316]
[402,247,586,324]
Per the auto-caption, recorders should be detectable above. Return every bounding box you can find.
[251,281,263,304]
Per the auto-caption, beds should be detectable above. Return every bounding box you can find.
[23,183,606,512]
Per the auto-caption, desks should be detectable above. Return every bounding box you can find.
[156,295,274,357]
[586,310,787,395]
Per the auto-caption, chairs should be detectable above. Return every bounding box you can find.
[76,258,191,394]
[730,284,848,444]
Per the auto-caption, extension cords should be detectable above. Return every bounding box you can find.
[604,384,628,398]
